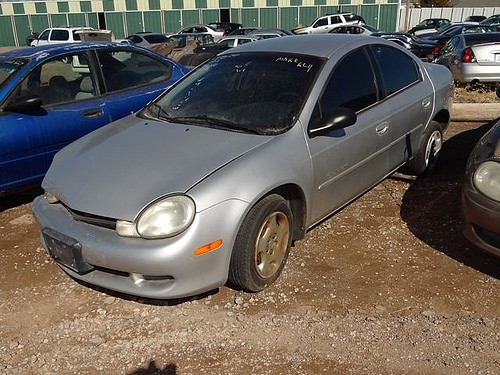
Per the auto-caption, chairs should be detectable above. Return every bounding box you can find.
[221,73,281,133]
[39,76,68,102]
[72,77,95,101]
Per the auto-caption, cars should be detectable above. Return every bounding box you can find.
[329,25,384,36]
[452,15,487,26]
[248,29,295,47]
[461,119,500,261]
[165,25,225,45]
[431,33,500,84]
[228,26,293,42]
[409,19,450,35]
[370,32,441,62]
[209,22,242,37]
[31,32,454,299]
[126,32,167,51]
[0,41,190,200]
[30,25,115,48]
[217,36,257,50]
[465,15,500,32]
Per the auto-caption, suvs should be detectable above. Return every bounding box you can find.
[295,12,365,34]
